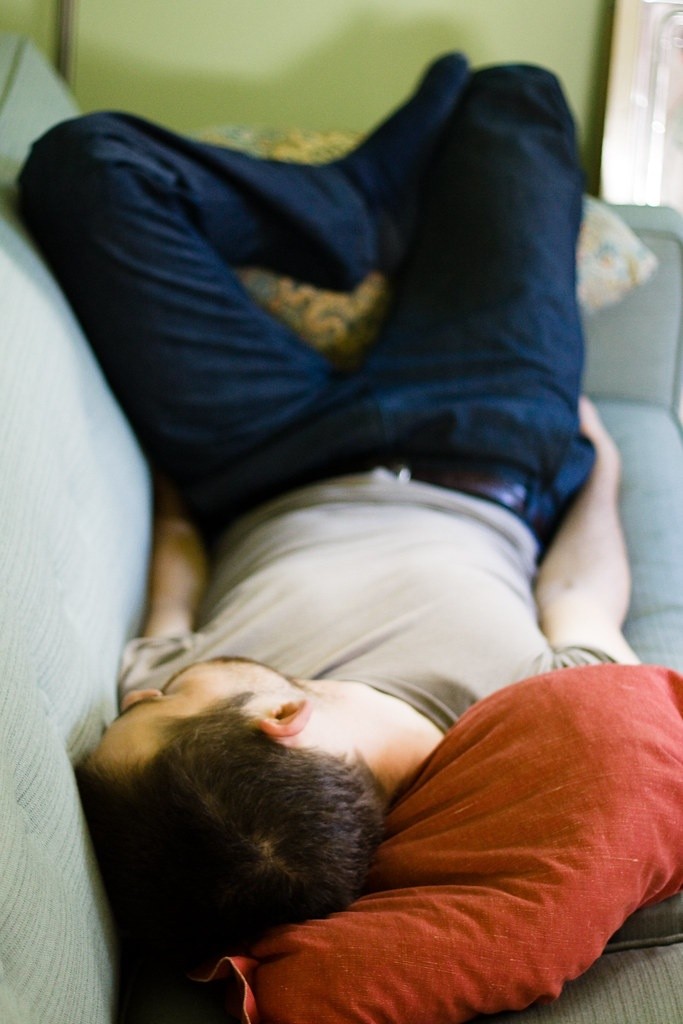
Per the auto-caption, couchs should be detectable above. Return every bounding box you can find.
[0,36,682,1024]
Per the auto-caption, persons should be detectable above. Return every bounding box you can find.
[16,52,642,954]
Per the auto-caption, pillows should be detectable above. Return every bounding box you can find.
[212,125,659,358]
[181,663,683,1024]
